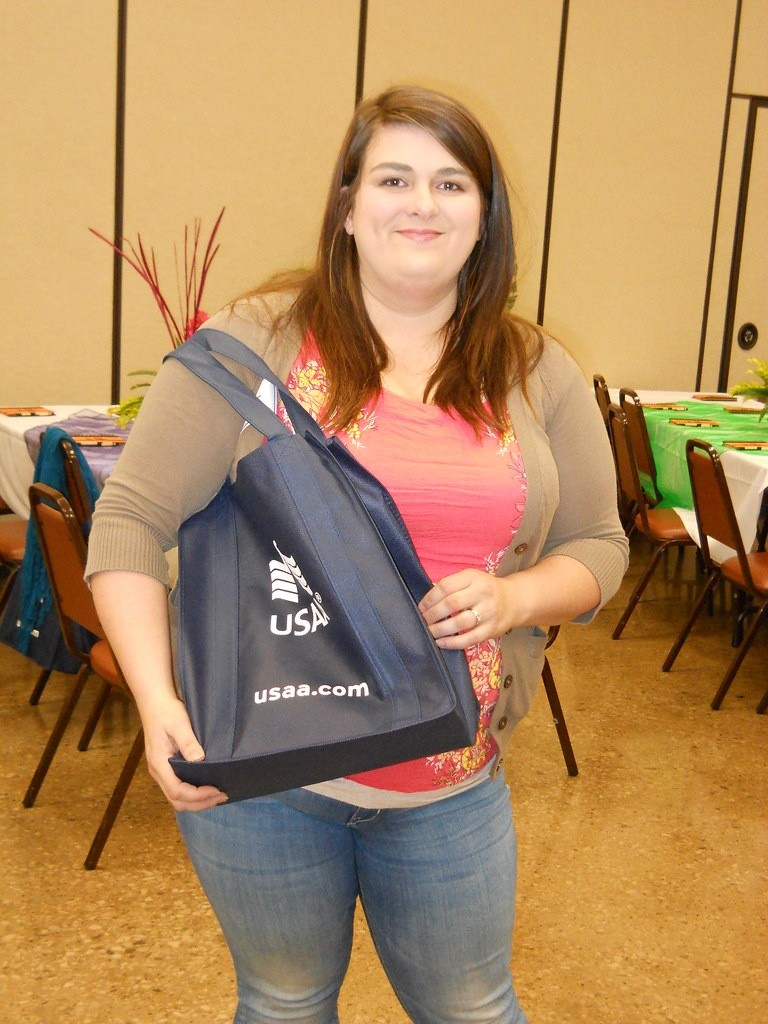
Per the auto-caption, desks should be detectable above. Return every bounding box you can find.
[0,404,179,590]
[608,388,768,649]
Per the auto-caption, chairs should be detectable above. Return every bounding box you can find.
[592,374,612,432]
[15,426,112,752]
[618,387,685,556]
[661,437,768,711]
[21,482,143,871]
[608,402,715,640]
[0,512,29,625]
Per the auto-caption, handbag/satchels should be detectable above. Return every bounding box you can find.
[164,329,482,806]
[0,567,95,675]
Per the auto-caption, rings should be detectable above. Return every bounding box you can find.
[467,609,480,625]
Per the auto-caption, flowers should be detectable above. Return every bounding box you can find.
[86,206,225,431]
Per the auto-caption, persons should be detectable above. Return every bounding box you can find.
[82,86,631,1024]
